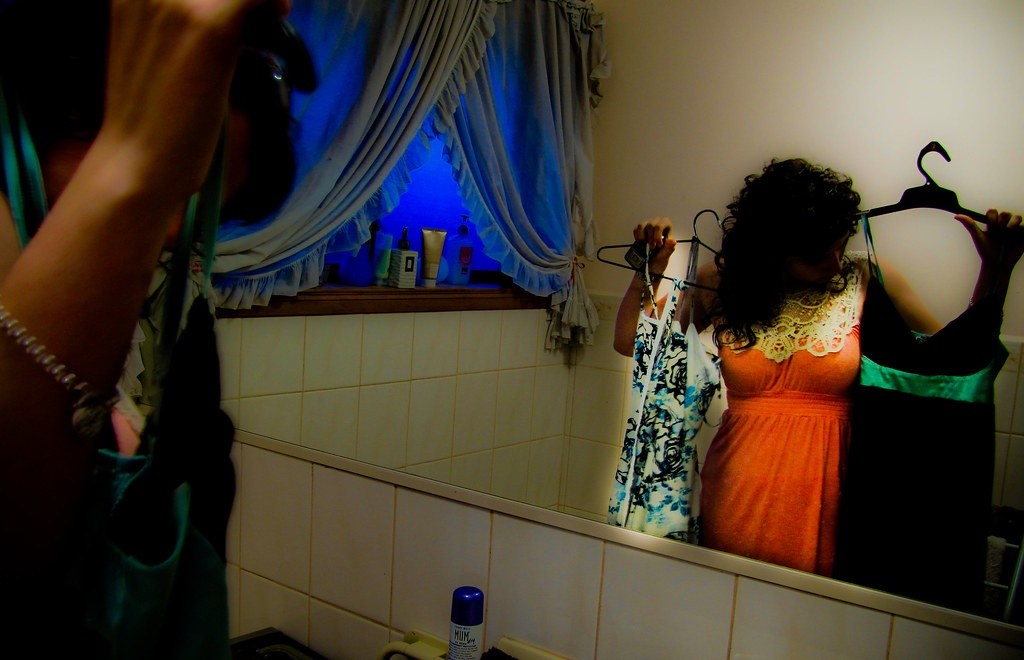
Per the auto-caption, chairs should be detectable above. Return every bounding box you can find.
[856,141,986,225]
[598,210,723,292]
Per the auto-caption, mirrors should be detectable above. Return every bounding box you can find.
[218,0,1024,648]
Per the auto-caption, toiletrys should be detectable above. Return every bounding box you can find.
[448,586,485,660]
[338,215,477,289]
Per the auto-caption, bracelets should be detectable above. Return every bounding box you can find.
[0,300,122,434]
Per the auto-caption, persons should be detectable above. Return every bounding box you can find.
[0,0,290,660]
[613,159,1024,578]
